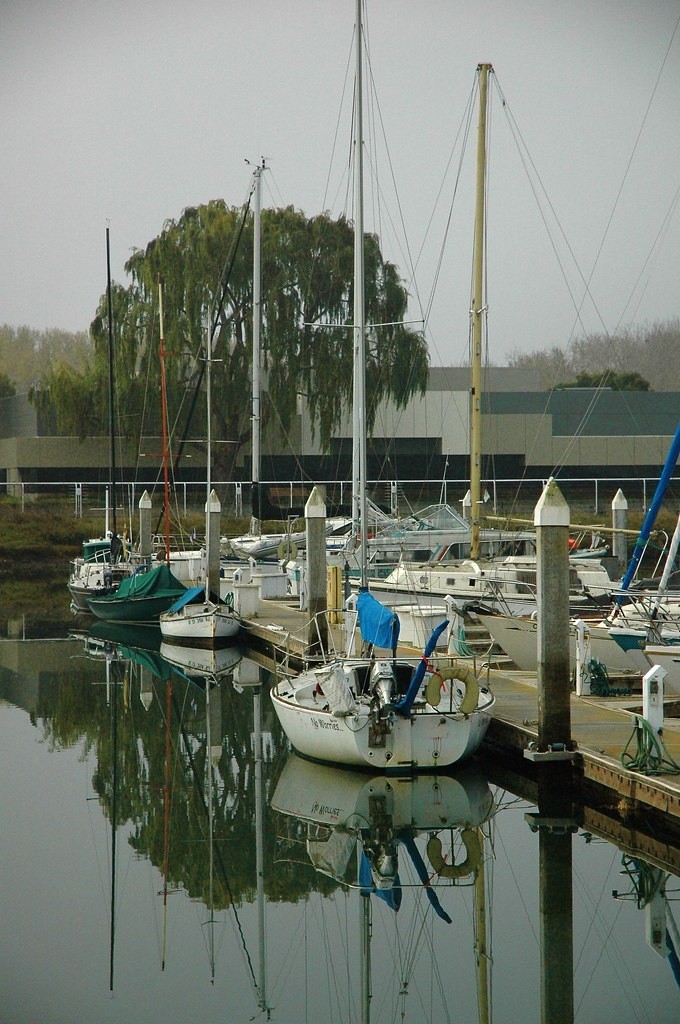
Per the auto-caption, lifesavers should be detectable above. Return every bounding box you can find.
[278,541,298,559]
[427,829,482,878]
[426,666,480,714]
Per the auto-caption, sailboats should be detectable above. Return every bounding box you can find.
[247,0,678,767]
[66,225,145,611]
[267,746,496,1024]
[160,304,241,640]
[87,282,184,622]
[79,616,245,996]
